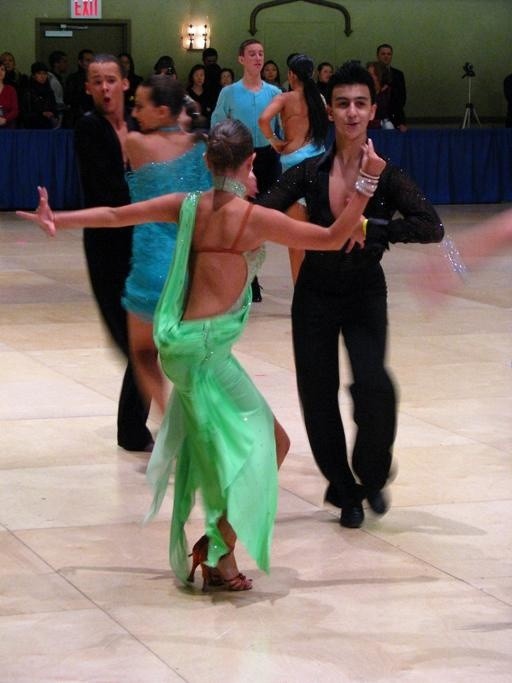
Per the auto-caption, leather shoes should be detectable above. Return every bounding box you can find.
[366,488,386,513]
[340,506,364,526]
[252,274,263,302]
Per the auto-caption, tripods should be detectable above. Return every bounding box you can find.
[461,77,482,129]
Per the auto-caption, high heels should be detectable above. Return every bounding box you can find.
[186,537,253,590]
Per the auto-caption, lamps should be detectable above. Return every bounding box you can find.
[181,22,211,51]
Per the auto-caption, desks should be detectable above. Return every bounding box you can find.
[321,125,512,203]
[0,127,90,211]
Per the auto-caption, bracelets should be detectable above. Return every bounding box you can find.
[362,218,368,241]
[354,168,380,198]
[265,133,280,145]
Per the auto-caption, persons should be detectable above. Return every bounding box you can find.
[503,74,512,128]
[245,61,445,528]
[120,73,214,407]
[210,38,286,302]
[14,118,386,596]
[411,209,512,299]
[260,45,405,131]
[69,54,158,453]
[257,52,328,291]
[117,46,235,129]
[1,47,95,128]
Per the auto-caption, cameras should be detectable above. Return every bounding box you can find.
[462,62,475,79]
